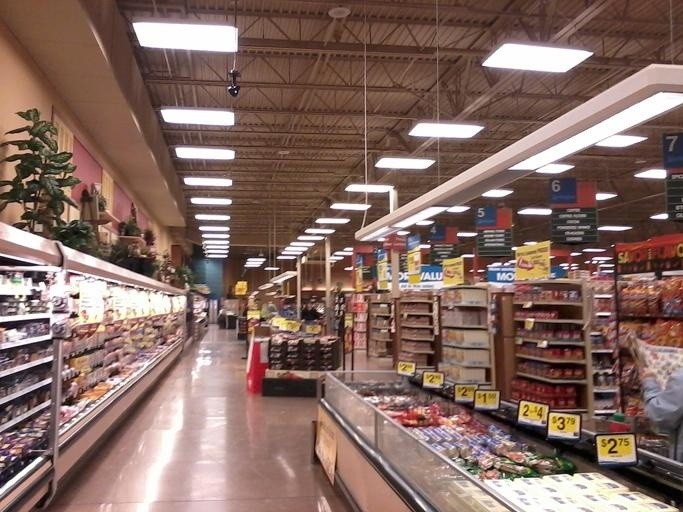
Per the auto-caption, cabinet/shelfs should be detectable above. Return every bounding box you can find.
[312,234,683,510]
[215,287,354,395]
[0,221,208,512]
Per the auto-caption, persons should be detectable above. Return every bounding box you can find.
[202,287,346,342]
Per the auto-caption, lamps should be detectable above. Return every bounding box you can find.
[277,255,296,259]
[191,198,233,206]
[298,236,326,242]
[482,44,594,74]
[597,135,648,147]
[196,214,230,218]
[519,208,551,216]
[204,240,230,259]
[282,250,304,256]
[634,169,669,180]
[162,106,235,127]
[536,165,575,175]
[290,241,316,247]
[315,220,351,224]
[185,178,232,187]
[594,192,619,200]
[175,147,234,160]
[202,234,230,239]
[483,189,516,197]
[409,122,484,138]
[245,258,267,269]
[198,226,230,232]
[133,21,243,54]
[376,158,435,169]
[345,184,394,193]
[456,233,478,237]
[284,245,308,251]
[377,206,472,235]
[331,203,372,211]
